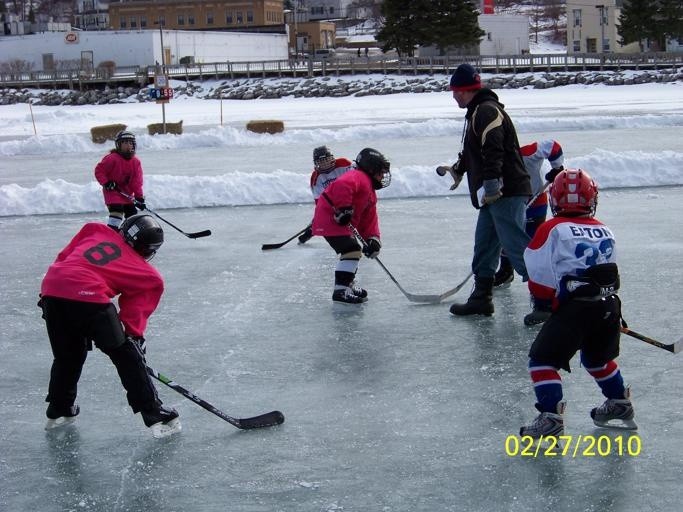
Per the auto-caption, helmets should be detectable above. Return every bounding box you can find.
[119,213,164,261]
[312,145,336,173]
[549,168,599,218]
[115,130,137,159]
[355,147,391,190]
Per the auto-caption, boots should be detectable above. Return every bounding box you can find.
[590,383,634,421]
[519,401,567,440]
[524,304,553,325]
[450,275,496,315]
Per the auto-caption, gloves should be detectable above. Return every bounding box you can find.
[105,180,118,191]
[134,196,147,211]
[334,205,353,226]
[362,238,382,260]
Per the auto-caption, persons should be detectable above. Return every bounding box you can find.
[37,214,180,427]
[494,138,564,286]
[95,130,145,231]
[520,167,634,439]
[298,146,352,243]
[310,148,392,305]
[444,64,553,326]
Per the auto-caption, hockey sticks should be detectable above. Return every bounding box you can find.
[116,189,212,238]
[262,223,312,250]
[145,366,285,429]
[621,326,683,353]
[432,181,551,304]
[321,191,440,304]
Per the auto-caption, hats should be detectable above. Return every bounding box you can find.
[449,63,483,92]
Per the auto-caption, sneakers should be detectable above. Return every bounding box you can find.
[46,401,81,420]
[142,401,179,427]
[332,284,367,304]
[494,269,514,285]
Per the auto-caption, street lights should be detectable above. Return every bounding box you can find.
[595,5,605,53]
[283,9,291,24]
[154,20,165,76]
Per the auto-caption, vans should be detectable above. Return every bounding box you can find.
[315,48,334,63]
